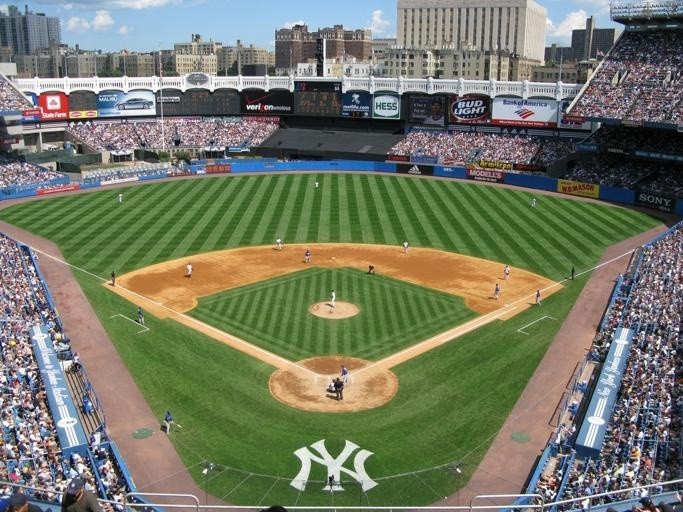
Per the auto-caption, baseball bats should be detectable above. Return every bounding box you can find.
[172,423,183,428]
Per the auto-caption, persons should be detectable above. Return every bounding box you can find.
[0,338,132,511]
[326,377,336,392]
[311,180,320,192]
[0,75,37,111]
[556,127,681,196]
[340,363,347,384]
[571,265,576,279]
[110,270,116,286]
[0,73,281,191]
[0,234,69,344]
[502,265,510,280]
[138,307,145,324]
[69,119,278,147]
[328,288,335,307]
[271,237,283,251]
[493,282,500,301]
[536,289,540,304]
[607,219,682,335]
[162,408,174,435]
[184,261,192,279]
[333,377,344,400]
[401,239,408,255]
[386,129,577,166]
[569,26,682,124]
[367,264,376,275]
[117,191,122,203]
[1,157,174,192]
[303,248,311,263]
[423,97,444,125]
[529,328,682,512]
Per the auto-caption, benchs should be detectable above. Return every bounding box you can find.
[0,69,44,115]
[562,26,681,201]
